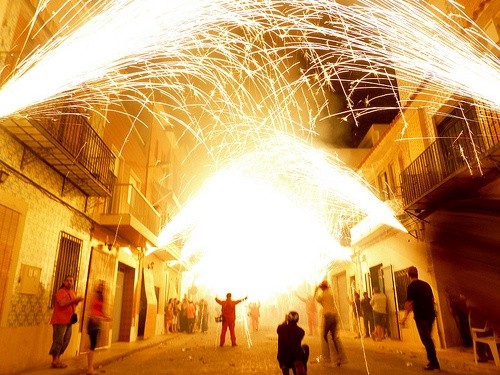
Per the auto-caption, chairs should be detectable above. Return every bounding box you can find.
[471,318,500,366]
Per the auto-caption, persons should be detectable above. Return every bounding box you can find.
[361,292,375,338]
[49,276,83,369]
[215,293,247,346]
[276,311,309,375]
[346,292,361,339]
[370,287,387,341]
[399,266,441,370]
[164,293,210,333]
[314,280,347,368]
